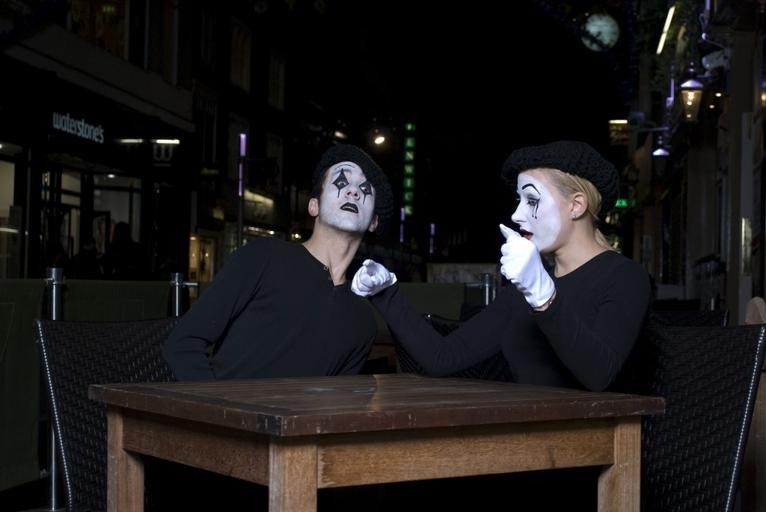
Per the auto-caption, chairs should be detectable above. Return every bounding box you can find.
[31,317,182,511]
[604,320,762,510]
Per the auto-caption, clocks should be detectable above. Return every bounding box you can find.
[580,14,620,53]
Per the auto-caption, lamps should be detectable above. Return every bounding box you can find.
[586,21,766,160]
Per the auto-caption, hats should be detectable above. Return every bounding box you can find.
[505,140,620,215]
[315,145,392,225]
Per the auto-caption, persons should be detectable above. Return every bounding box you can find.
[65,221,154,278]
[350,140,652,394]
[160,144,394,385]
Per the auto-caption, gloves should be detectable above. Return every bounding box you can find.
[499,223,555,307]
[351,258,399,296]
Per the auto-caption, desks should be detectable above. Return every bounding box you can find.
[87,370,665,511]
